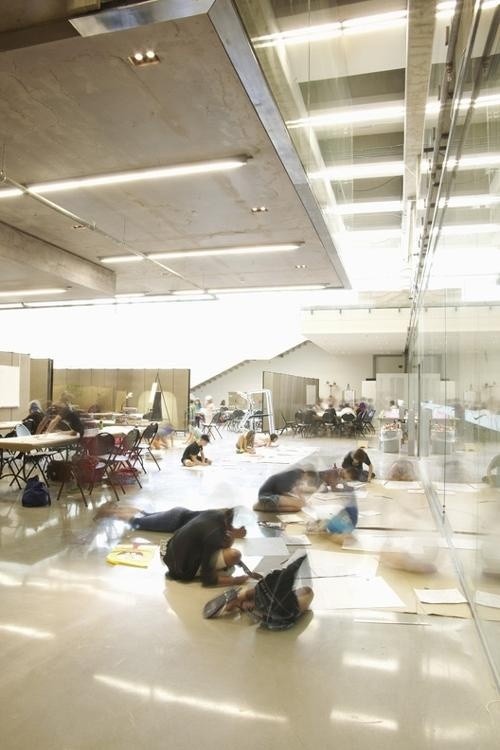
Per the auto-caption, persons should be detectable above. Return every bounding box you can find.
[6,394,376,631]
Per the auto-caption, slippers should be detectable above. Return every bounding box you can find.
[202,589,237,618]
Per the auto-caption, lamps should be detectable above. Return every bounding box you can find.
[2,155,249,199]
[111,270,331,300]
[96,216,307,266]
[3,286,73,299]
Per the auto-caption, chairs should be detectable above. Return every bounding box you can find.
[190,402,380,443]
[0,407,177,509]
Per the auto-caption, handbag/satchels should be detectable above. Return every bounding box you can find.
[22,475,51,507]
